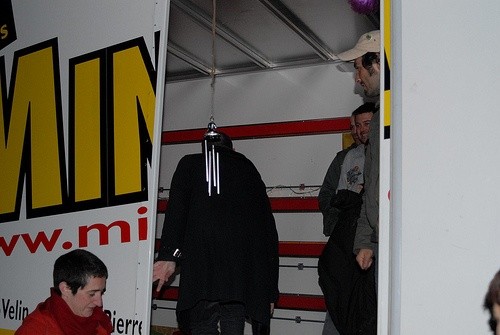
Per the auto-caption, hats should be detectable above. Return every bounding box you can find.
[337,30,380,62]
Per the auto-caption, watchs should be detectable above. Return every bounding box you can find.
[162,241,182,260]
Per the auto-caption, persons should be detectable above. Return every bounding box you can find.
[317,30,380,335]
[153,130,279,335]
[15,250,120,335]
[480,270,500,335]
